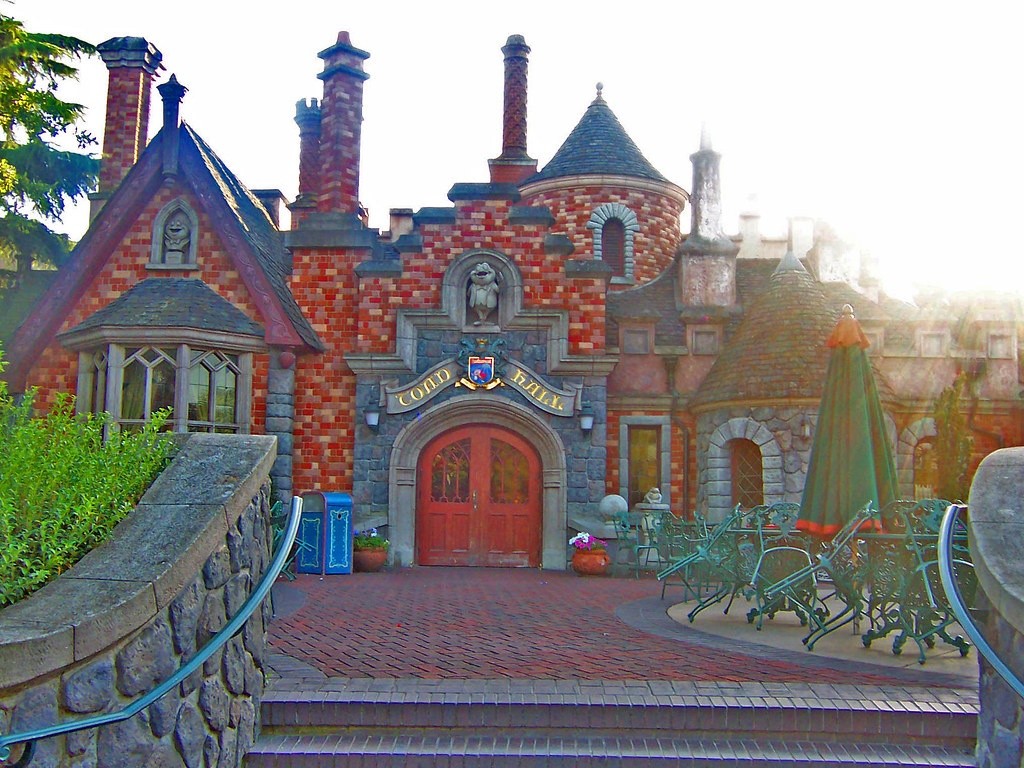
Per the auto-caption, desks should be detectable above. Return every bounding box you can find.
[725,530,825,625]
[854,534,971,657]
[654,521,739,603]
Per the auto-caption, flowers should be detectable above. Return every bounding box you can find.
[568,532,608,550]
[353,527,390,552]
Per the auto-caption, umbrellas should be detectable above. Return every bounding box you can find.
[795,318,905,597]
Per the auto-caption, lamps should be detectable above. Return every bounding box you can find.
[362,395,382,433]
[578,401,595,437]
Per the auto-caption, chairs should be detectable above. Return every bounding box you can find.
[269,500,317,582]
[611,498,978,664]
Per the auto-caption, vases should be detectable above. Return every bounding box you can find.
[353,547,388,572]
[572,549,611,578]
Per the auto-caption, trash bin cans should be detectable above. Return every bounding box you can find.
[296,491,353,575]
